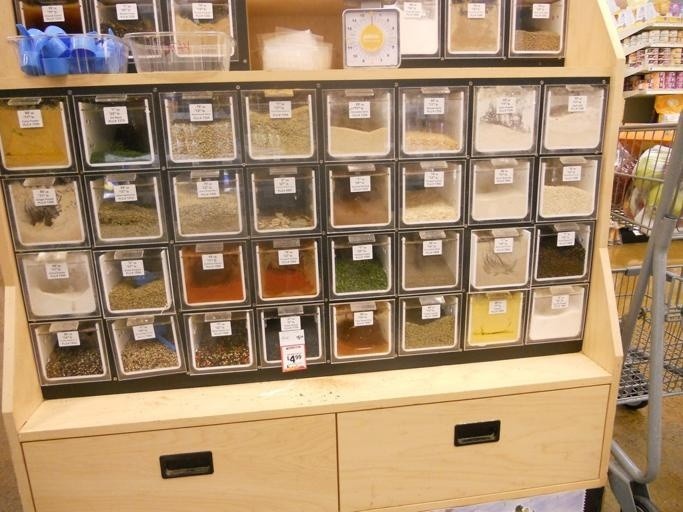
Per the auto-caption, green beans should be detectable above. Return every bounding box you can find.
[44,252,455,378]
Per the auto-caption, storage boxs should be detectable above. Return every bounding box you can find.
[0,0,610,400]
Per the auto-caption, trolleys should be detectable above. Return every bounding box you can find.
[606,107,683,510]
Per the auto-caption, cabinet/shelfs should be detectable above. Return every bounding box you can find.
[338,384,610,512]
[22,411,339,512]
